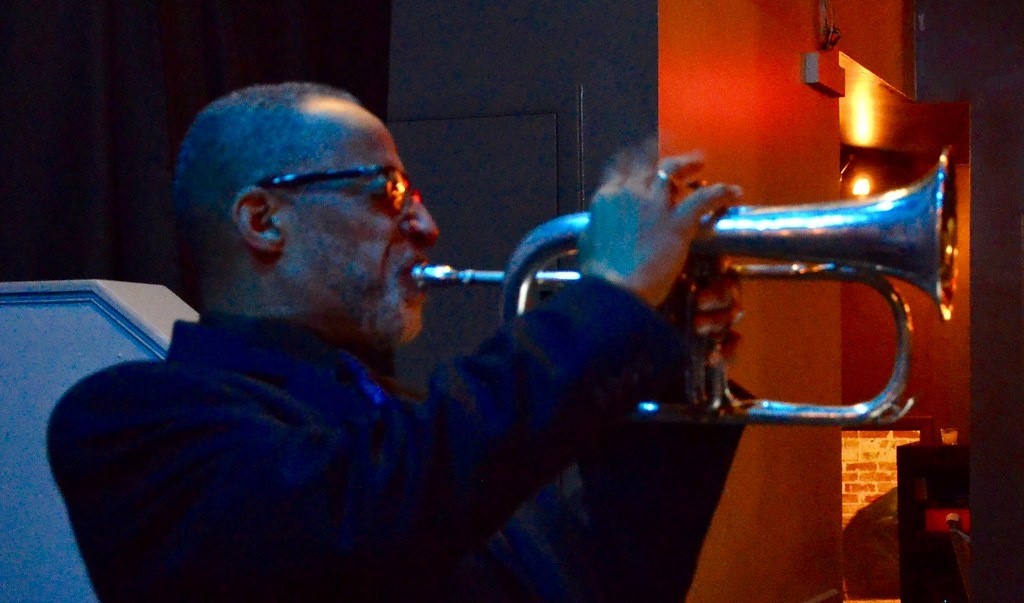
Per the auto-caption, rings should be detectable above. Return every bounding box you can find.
[656,169,671,182]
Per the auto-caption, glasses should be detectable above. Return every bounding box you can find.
[253,165,425,215]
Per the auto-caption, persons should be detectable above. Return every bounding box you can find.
[45,82,762,603]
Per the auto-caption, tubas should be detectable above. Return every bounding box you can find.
[413,143,959,427]
